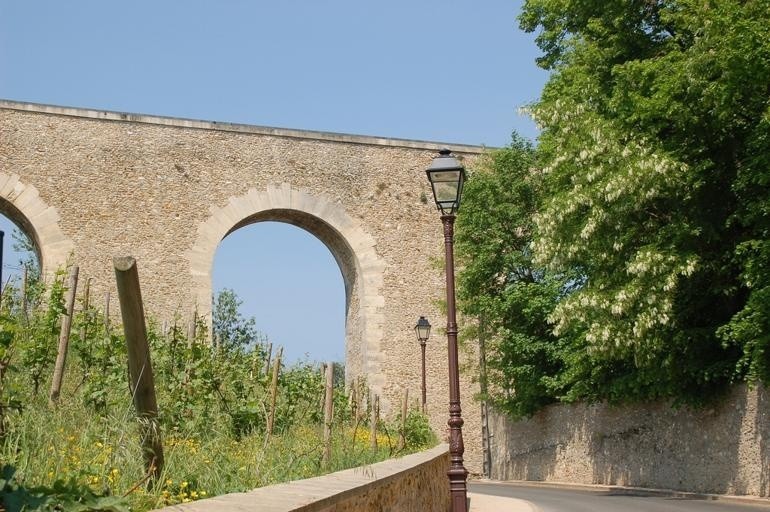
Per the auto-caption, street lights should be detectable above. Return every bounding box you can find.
[413,315,431,417]
[425,145,467,510]
